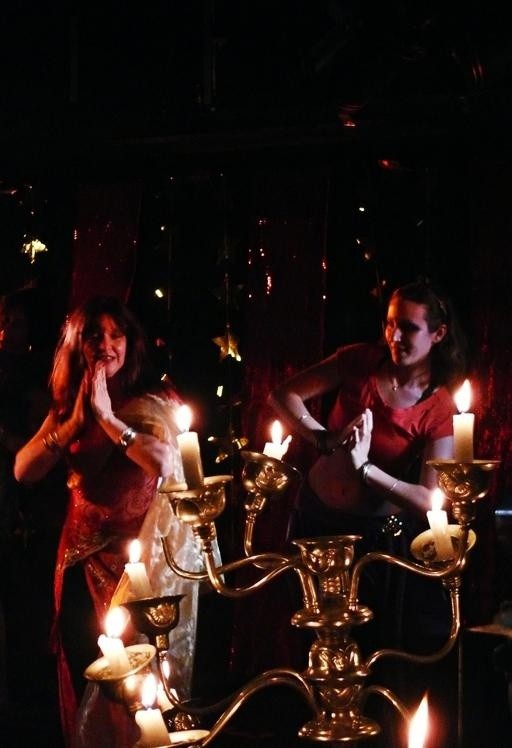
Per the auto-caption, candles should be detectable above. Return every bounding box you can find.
[261,423,287,461]
[177,402,201,486]
[125,539,152,601]
[405,688,433,748]
[96,611,131,670]
[132,680,170,744]
[424,487,457,561]
[450,379,478,462]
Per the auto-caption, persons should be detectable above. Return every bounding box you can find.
[2,279,459,748]
